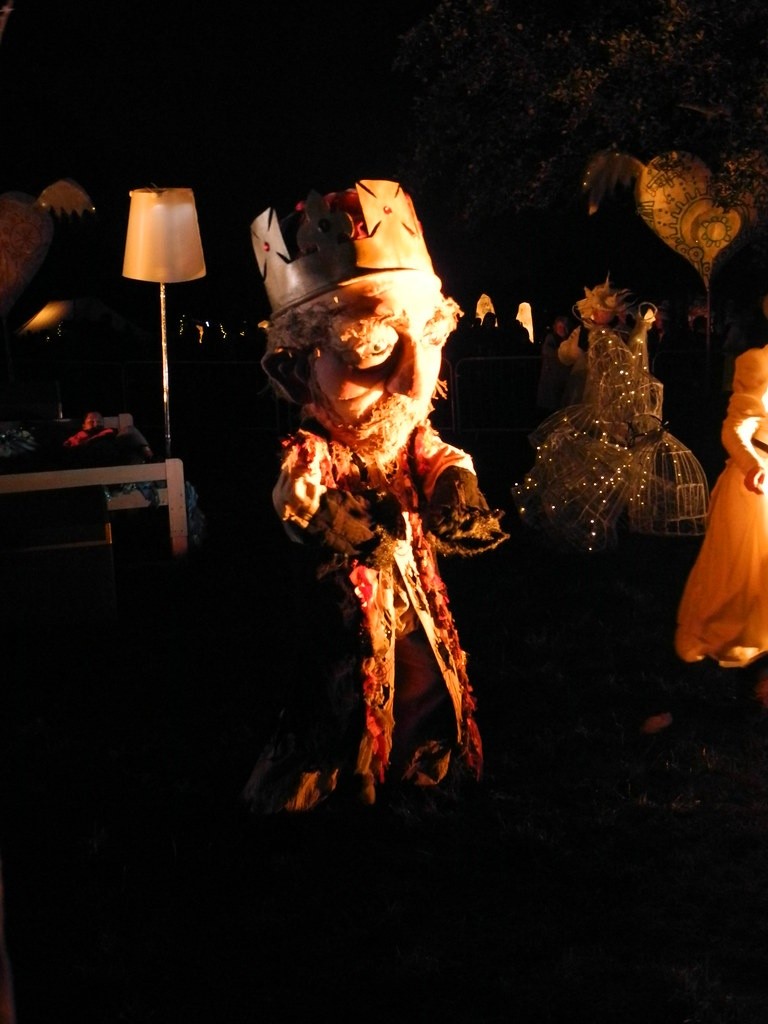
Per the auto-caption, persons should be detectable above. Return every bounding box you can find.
[674,296,768,668]
[234,179,501,810]
[508,278,711,543]
[62,411,116,450]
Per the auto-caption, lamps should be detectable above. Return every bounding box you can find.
[122,184,205,460]
